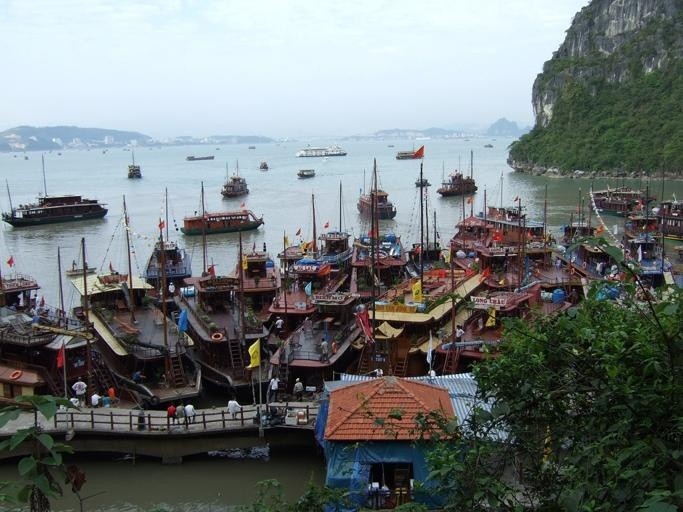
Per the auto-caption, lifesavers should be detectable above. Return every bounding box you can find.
[211,333,223,340]
[332,342,338,353]
[10,370,23,380]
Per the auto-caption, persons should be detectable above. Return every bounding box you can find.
[72,376,118,409]
[169,282,175,298]
[274,316,284,338]
[455,325,465,342]
[293,378,304,402]
[132,369,145,384]
[268,375,280,403]
[167,401,197,425]
[568,288,579,305]
[320,338,331,364]
[227,395,242,421]
[554,257,561,272]
[372,366,383,377]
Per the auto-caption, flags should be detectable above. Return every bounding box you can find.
[56,339,65,368]
[245,338,262,370]
[426,330,433,364]
[7,255,15,268]
[493,232,503,242]
[410,144,424,160]
[479,266,492,283]
[356,310,375,344]
[587,184,625,256]
[229,193,465,303]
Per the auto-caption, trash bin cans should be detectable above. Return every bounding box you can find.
[138,413,146,431]
